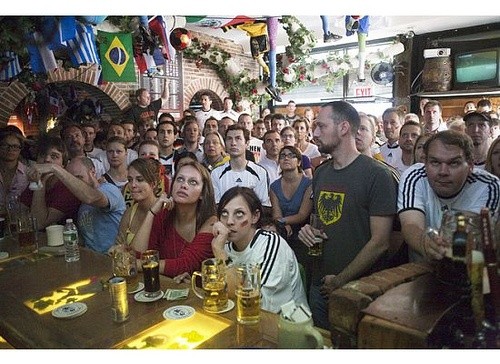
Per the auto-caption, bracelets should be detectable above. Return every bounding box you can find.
[280,218,287,224]
[224,257,234,269]
[150,208,157,216]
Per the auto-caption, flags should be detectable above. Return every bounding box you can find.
[25,79,59,129]
[0,15,100,80]
[96,16,174,86]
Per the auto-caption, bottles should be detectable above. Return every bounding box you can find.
[62,218,80,262]
[448,208,500,349]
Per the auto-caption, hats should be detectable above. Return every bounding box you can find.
[463,110,491,122]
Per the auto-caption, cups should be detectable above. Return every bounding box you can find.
[307,212,324,256]
[111,245,139,291]
[140,250,161,298]
[18,217,39,264]
[277,312,324,349]
[46,225,64,247]
[234,260,262,325]
[427,207,479,297]
[190,257,229,312]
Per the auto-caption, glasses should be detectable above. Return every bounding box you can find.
[279,153,297,159]
[0,141,21,151]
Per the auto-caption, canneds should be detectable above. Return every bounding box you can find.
[109,278,129,322]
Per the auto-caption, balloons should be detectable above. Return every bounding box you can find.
[170,28,191,50]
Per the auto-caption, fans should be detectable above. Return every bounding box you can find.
[371,62,396,85]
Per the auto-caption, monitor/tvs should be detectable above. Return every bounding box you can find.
[454,47,500,89]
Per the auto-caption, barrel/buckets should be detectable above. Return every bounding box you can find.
[423,57,453,92]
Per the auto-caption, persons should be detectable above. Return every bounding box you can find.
[447,99,500,179]
[109,155,218,276]
[297,101,394,331]
[396,130,500,262]
[124,74,169,130]
[173,186,310,316]
[320,15,343,43]
[135,92,331,261]
[266,16,282,102]
[0,120,136,255]
[250,35,270,81]
[345,15,369,82]
[355,98,447,232]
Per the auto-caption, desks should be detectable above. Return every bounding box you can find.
[0,229,333,349]
